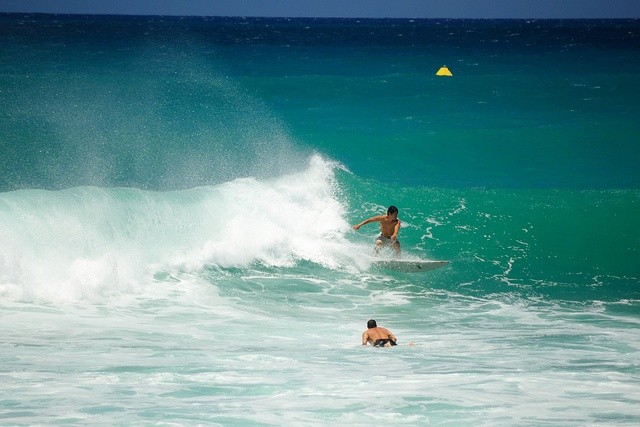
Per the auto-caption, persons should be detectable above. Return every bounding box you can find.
[352,206,402,259]
[362,318,416,349]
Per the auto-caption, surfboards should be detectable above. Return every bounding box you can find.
[377,258,449,273]
